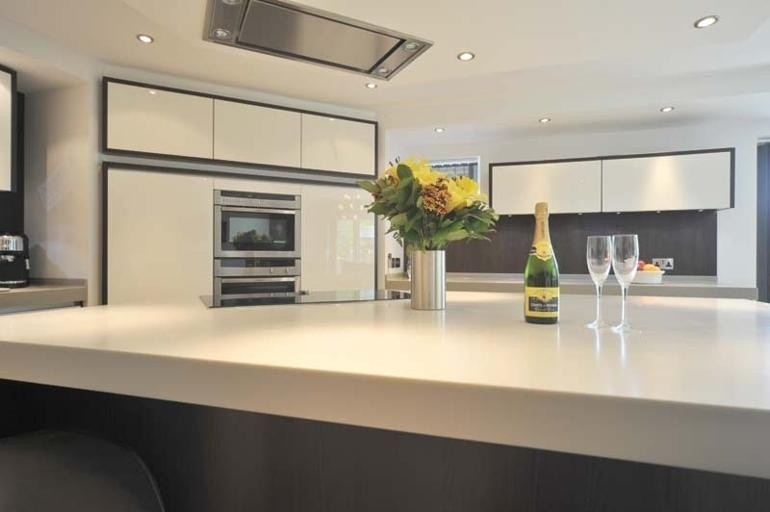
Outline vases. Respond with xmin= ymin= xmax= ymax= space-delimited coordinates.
xmin=412 ymin=250 xmax=446 ymax=310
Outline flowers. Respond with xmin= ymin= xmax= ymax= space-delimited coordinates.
xmin=360 ymin=156 xmax=499 ymax=248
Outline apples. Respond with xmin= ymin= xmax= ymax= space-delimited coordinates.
xmin=636 ymin=260 xmax=644 ymax=270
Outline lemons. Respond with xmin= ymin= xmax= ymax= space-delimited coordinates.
xmin=642 ymin=263 xmax=660 ymax=272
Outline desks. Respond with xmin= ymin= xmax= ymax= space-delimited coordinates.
xmin=0 ymin=280 xmax=88 ymax=311
xmin=386 ymin=269 xmax=716 ymax=296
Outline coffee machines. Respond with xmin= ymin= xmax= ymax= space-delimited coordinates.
xmin=0 ymin=232 xmax=31 ymax=288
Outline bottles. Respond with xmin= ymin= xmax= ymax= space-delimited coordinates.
xmin=525 ymin=202 xmax=561 ymax=322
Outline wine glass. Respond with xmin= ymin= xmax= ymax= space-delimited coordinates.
xmin=584 ymin=235 xmax=611 ymax=328
xmin=611 ymin=234 xmax=639 ymax=331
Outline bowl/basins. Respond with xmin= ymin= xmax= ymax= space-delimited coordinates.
xmin=633 ymin=270 xmax=665 ymax=285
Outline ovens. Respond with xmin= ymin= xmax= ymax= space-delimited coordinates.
xmin=213 ymin=189 xmax=309 ymax=305
